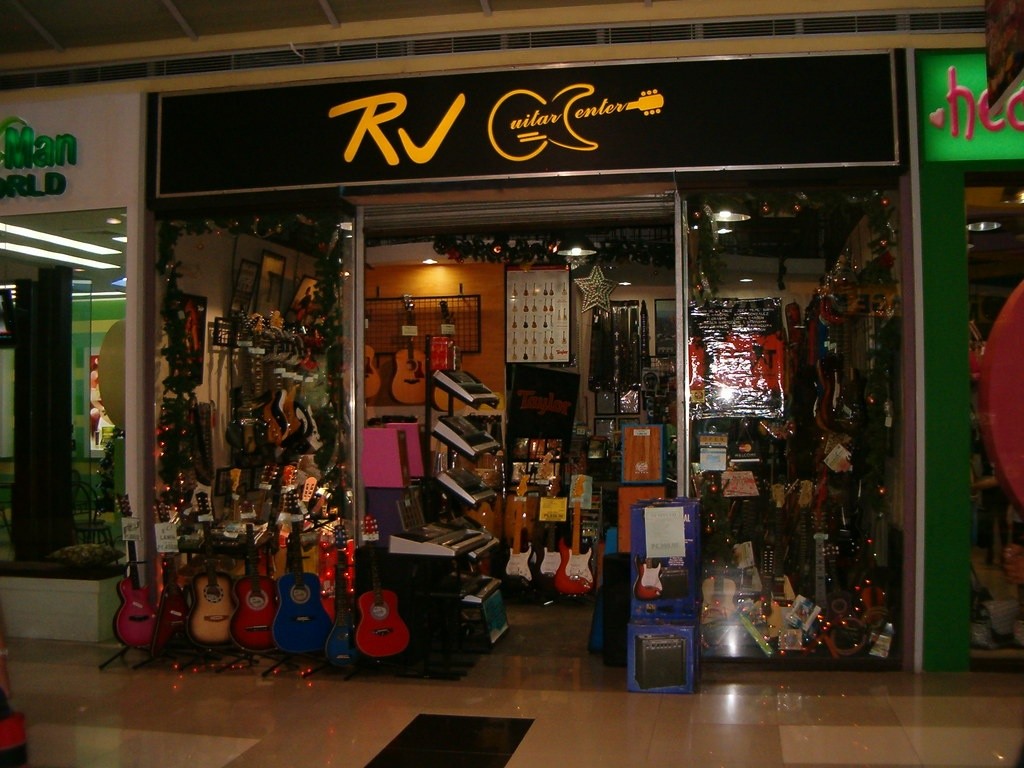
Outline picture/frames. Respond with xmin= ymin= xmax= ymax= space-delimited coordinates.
xmin=228 ymin=250 xmax=319 ymax=315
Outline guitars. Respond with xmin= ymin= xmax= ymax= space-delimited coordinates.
xmin=110 ymin=488 xmax=412 ymax=674
xmin=224 ymin=308 xmax=331 ymax=471
xmin=500 ymin=445 xmax=597 ymax=598
xmin=770 ymin=214 xmax=900 ymax=661
xmin=632 ymin=551 xmax=668 ymax=602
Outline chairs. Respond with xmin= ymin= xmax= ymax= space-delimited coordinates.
xmin=1 ymin=468 xmax=122 ymax=565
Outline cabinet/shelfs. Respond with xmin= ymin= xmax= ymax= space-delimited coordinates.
xmin=387 ymin=338 xmax=504 ymax=682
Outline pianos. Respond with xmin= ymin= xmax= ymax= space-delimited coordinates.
xmin=383 ymin=366 xmax=504 ymax=566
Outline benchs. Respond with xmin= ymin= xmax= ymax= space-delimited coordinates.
xmin=0 ymin=560 xmax=125 ymax=643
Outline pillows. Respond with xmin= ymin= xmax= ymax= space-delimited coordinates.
xmin=46 ymin=544 xmax=125 ymax=571
